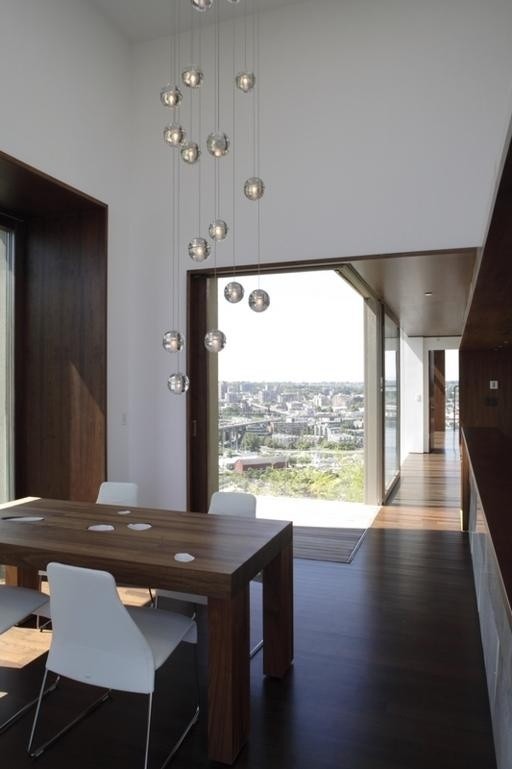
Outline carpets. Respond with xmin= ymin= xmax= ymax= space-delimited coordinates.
xmin=256 ymin=497 xmax=382 ymax=564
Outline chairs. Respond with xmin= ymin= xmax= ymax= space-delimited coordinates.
xmin=27 ymin=482 xmax=155 ymax=631
xmin=1 ymin=582 xmax=61 ymax=733
xmin=153 ymin=492 xmax=263 ymax=660
xmin=26 ymin=559 xmax=198 ymax=769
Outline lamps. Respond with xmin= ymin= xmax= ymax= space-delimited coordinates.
xmin=159 ymin=1 xmax=269 ymax=393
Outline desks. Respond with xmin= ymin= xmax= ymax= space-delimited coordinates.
xmin=0 ymin=496 xmax=292 ymax=765
